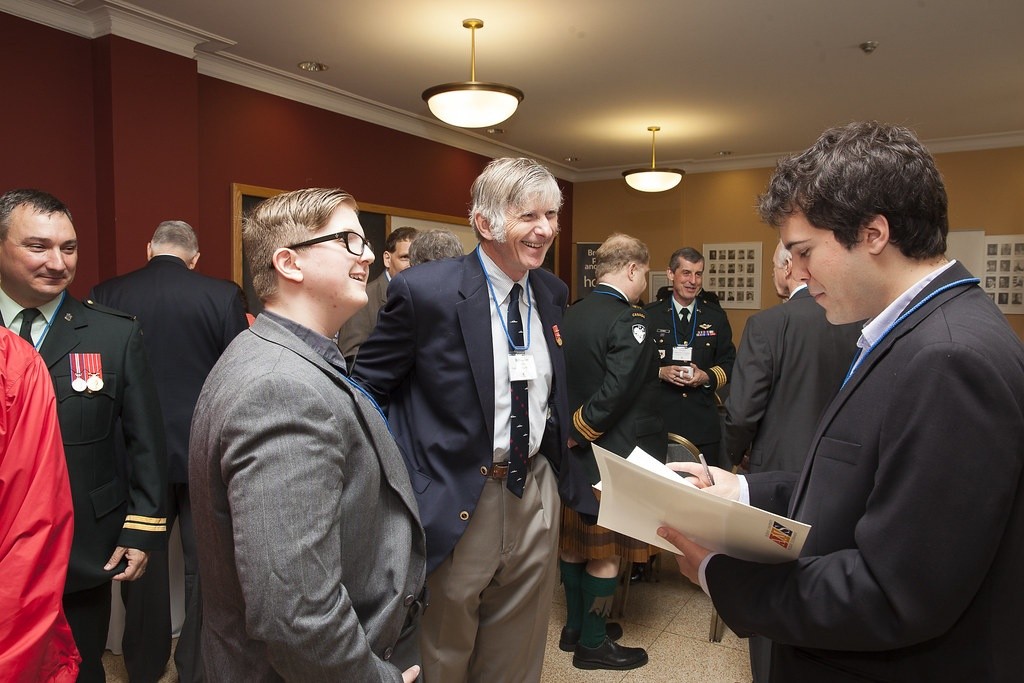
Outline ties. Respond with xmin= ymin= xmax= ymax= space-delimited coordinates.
xmin=680 ymin=307 xmax=691 ymax=342
xmin=507 ymin=284 xmax=529 ymax=500
xmin=19 ymin=308 xmax=41 ymax=347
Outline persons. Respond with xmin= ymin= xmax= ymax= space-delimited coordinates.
xmin=0 ymin=328 xmax=86 ymax=683
xmin=655 ymin=120 xmax=1024 ymax=683
xmin=556 ymin=232 xmax=661 ymax=671
xmin=630 ymin=247 xmax=735 ymax=583
xmin=709 ymin=250 xmax=755 ymax=301
xmin=337 ymin=226 xmax=465 ymax=356
xmin=349 ymin=157 xmax=569 ymax=683
xmin=174 ymin=186 xmax=428 ymax=683
xmin=985 ymin=243 xmax=1024 ymax=304
xmin=88 ymin=219 xmax=250 ymax=683
xmin=0 ymin=189 xmax=179 ymax=683
xmin=723 ymin=237 xmax=871 ymax=473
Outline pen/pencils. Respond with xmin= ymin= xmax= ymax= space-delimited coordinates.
xmin=698 ymin=454 xmax=716 ymax=486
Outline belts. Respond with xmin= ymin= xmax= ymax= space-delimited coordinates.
xmin=489 ymin=452 xmax=541 ymax=480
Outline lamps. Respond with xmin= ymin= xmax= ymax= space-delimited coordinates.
xmin=622 ymin=126 xmax=686 ymax=193
xmin=422 ymin=17 xmax=525 ymax=129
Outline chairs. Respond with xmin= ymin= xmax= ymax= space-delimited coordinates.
xmin=620 ymin=428 xmax=725 ymax=644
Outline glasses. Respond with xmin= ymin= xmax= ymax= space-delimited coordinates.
xmin=269 ymin=230 xmax=371 ymax=269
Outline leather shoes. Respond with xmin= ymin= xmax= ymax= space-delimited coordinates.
xmin=572 ymin=636 xmax=648 ymax=670
xmin=559 ymin=622 xmax=623 ymax=652
xmin=623 ymin=553 xmax=656 ymax=582
